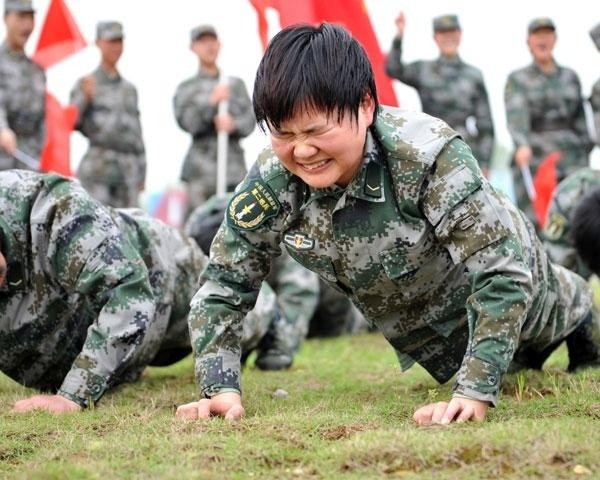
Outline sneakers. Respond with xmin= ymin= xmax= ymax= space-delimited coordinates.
xmin=256 ymin=308 xmax=297 ymax=370
xmin=568 ymin=310 xmax=600 ymax=376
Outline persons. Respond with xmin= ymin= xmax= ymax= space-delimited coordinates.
xmin=505 ymin=17 xmax=593 ymax=239
xmin=68 ymin=21 xmax=146 ymax=208
xmin=175 ymin=23 xmax=600 ymax=425
xmin=385 ymin=11 xmax=493 ymax=182
xmin=175 ymin=26 xmax=256 ymax=215
xmin=540 ymin=169 xmax=600 ymax=283
xmin=0 ymin=169 xmax=293 ymax=415
xmin=187 ymin=194 xmax=373 ymax=343
xmin=0 ymin=0 xmax=48 ymax=169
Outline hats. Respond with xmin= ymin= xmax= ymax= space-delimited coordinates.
xmin=190 ymin=25 xmax=217 ymax=42
xmin=2 ymin=0 xmax=35 ymax=15
xmin=96 ymin=22 xmax=124 ymax=42
xmin=433 ymin=15 xmax=460 ymax=33
xmin=529 ymin=18 xmax=554 ymax=33
